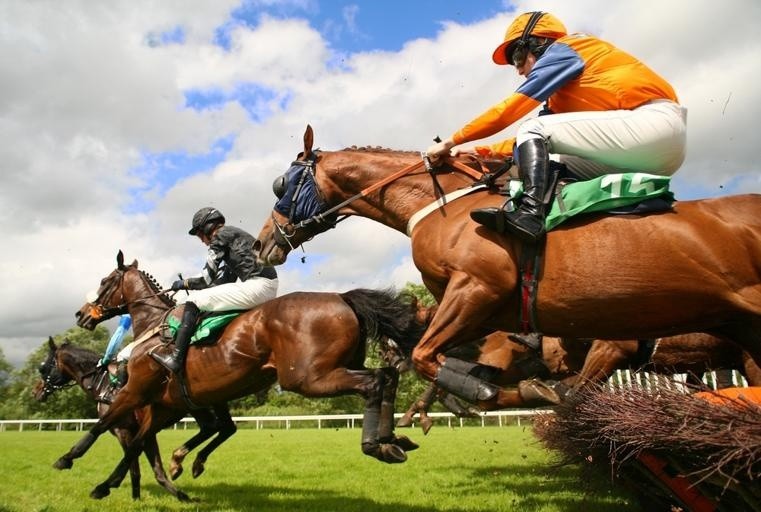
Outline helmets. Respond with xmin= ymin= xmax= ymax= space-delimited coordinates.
xmin=189 ymin=208 xmax=225 ymax=235
xmin=493 ymin=12 xmax=565 ymax=65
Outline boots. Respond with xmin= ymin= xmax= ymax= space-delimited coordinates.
xmin=96 ymin=362 xmax=127 ymax=401
xmin=471 ymin=139 xmax=549 ymax=242
xmin=148 ymin=311 xmax=197 ymax=375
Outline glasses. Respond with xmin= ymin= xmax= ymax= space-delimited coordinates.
xmin=513 ymin=12 xmax=542 ymax=67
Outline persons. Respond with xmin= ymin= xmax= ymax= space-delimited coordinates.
xmin=422 ymin=12 xmax=691 ymax=242
xmin=95 ymin=314 xmax=133 ymax=387
xmin=506 ymin=332 xmax=542 ymax=353
xmin=147 ymin=205 xmax=279 ymax=376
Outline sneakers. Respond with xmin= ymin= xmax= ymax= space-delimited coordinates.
xmin=507 ymin=333 xmax=542 ymax=351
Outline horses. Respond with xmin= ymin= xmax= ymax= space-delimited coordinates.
xmin=31 ymin=335 xmax=270 ymax=480
xmin=50 ymin=248 xmax=433 ymax=499
xmin=250 ymin=122 xmax=760 ymax=435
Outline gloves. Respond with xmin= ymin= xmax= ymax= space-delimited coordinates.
xmin=172 ymin=278 xmax=190 ymax=291
